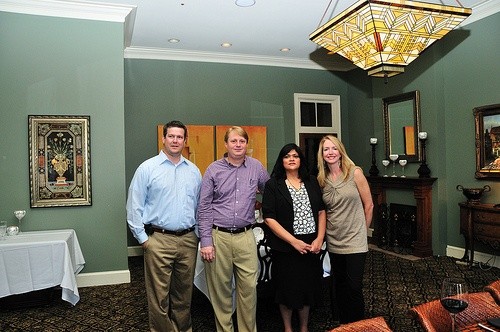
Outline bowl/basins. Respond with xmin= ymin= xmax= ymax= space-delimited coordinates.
xmin=6 ymin=226 xmax=19 ymax=236
xmin=457 ymin=184 xmax=490 ymax=204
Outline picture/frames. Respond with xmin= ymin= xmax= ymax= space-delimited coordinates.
xmin=27 ymin=114 xmax=93 ymax=209
xmin=382 ymin=90 xmax=424 ymax=164
xmin=472 ymin=103 xmax=500 ymax=182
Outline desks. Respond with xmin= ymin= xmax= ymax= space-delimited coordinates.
xmin=366 ymin=175 xmax=437 ymax=258
xmin=0 ymin=228 xmax=86 ymax=306
xmin=458 ymin=202 xmax=500 ymax=270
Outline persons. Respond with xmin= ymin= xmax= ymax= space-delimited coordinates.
xmin=262 ymin=143 xmax=326 ymax=332
xmin=126 ymin=121 xmax=202 ymax=332
xmin=198 ymin=125 xmax=270 ymax=332
xmin=317 ymin=135 xmax=374 ymax=327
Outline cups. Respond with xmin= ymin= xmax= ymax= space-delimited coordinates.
xmin=0 ymin=221 xmax=7 ymax=240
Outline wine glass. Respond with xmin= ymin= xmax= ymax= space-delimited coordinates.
xmin=14 ymin=210 xmax=26 ymax=236
xmin=382 ymin=155 xmax=406 ymax=178
xmin=441 ymin=277 xmax=469 ymax=332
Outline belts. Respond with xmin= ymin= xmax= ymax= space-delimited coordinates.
xmin=212 ymin=224 xmax=252 ymax=234
xmin=151 ymin=227 xmax=195 ymax=236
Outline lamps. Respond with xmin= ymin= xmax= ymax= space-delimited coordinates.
xmin=308 ymin=0 xmax=473 ymax=85
